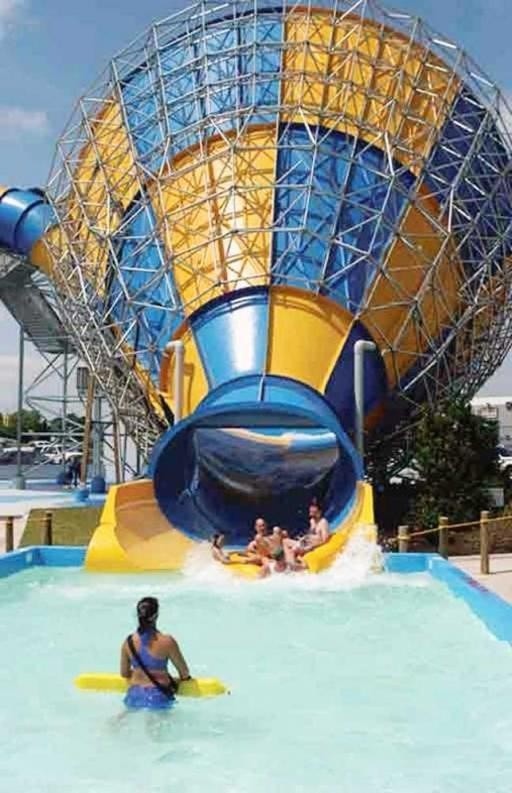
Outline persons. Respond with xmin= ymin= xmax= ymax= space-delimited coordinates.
xmin=208 ymin=531 xmax=262 ymax=566
xmin=247 ymin=496 xmax=331 ymax=575
xmin=119 ymin=595 xmax=191 ymax=711
